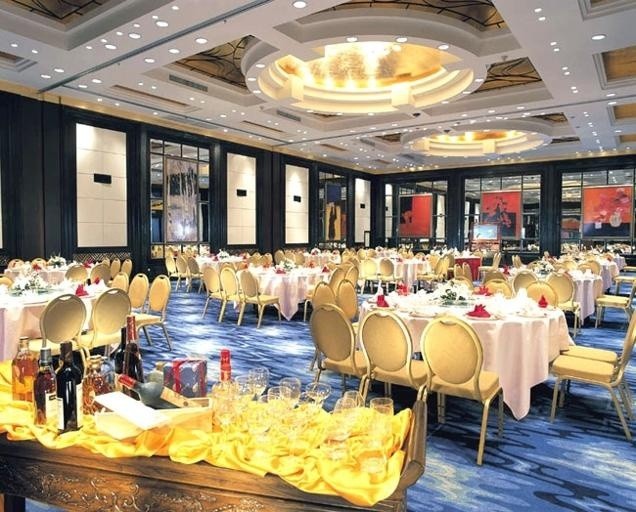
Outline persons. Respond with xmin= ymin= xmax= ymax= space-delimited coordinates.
xmin=325 ymin=205 xmax=338 ymax=241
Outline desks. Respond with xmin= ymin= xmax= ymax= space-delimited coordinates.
xmin=0 ymin=358 xmax=429 ymax=511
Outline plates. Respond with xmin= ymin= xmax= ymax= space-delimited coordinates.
xmin=368 ymin=292 xmax=556 ymax=321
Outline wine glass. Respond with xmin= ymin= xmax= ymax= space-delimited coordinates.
xmin=210 ymin=366 xmax=397 ymax=459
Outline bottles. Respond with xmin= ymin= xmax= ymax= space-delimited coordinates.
xmin=219 ymin=348 xmax=232 ymax=383
xmin=114 ymin=316 xmax=143 ymax=400
xmin=13 ymin=335 xmax=111 ymax=434
xmin=117 ymin=372 xmax=204 ymax=411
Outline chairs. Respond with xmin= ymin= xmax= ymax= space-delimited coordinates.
xmin=0 ymin=256 xmax=173 ymax=369
